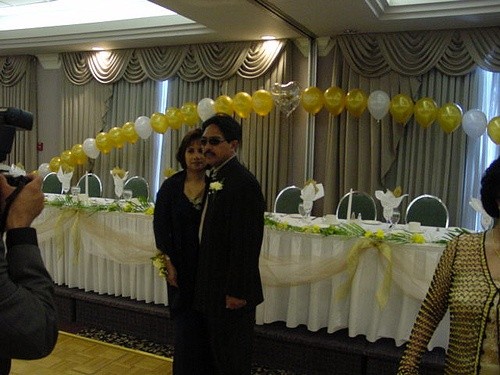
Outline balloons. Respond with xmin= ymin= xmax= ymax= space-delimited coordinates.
xmin=29 ymin=80 xmax=500 ymax=177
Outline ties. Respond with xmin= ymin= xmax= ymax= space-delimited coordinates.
xmin=199 ymin=170 xmax=214 ymax=246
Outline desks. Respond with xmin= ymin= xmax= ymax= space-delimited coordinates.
xmin=31 ymin=193 xmax=476 ymax=354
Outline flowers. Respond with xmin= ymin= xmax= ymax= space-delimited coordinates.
xmin=150 ymin=253 xmax=168 ymax=277
xmin=210 ymin=181 xmax=223 ymax=195
xmin=44 ymin=196 xmax=472 ymax=245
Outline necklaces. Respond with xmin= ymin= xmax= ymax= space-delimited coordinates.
xmin=492 ymin=229 xmax=500 ymax=257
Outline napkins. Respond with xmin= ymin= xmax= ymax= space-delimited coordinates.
xmin=110 ymin=170 xmax=129 ymax=197
xmin=300 ymin=183 xmax=324 ymax=209
xmin=375 ymin=189 xmax=409 ymax=218
xmin=57 ymin=165 xmax=73 ymax=189
xmin=469 ymin=195 xmax=491 ymax=226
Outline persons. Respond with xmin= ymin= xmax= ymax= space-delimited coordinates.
xmin=397 ymin=157 xmax=500 ymax=375
xmin=151 ymin=111 xmax=266 ymax=375
xmin=0 ymin=171 xmax=59 ymax=375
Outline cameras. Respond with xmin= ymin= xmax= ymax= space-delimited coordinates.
xmin=0 ymin=106 xmax=33 ymax=187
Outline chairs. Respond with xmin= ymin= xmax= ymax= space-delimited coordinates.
xmin=123 ymin=176 xmax=149 ymax=201
xmin=274 ymin=185 xmax=303 ymax=215
xmin=77 ymin=173 xmax=102 ymax=198
xmin=41 ymin=172 xmax=63 ymax=194
xmin=405 ymin=194 xmax=450 ymax=228
xmin=336 ymin=191 xmax=377 ymax=221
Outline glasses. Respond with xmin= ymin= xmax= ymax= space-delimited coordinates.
xmin=200 ymin=137 xmax=226 ymax=146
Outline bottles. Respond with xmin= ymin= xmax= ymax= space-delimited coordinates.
xmin=352 ymin=211 xmax=361 ymax=221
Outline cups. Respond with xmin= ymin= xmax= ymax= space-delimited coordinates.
xmin=408 ymin=222 xmax=420 ymax=231
xmin=48 ymin=194 xmax=57 ymax=202
xmin=481 ymin=214 xmax=494 ymax=231
xmin=326 ymin=215 xmax=337 ymax=223
xmin=71 ymin=188 xmax=80 ymax=197
xmin=78 ymin=194 xmax=88 ymax=201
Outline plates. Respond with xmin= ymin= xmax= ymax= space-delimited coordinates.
xmin=404 ymin=226 xmax=425 ymax=233
xmin=362 ymin=221 xmax=382 ymax=224
xmin=322 ymin=220 xmax=343 ymax=225
xmin=271 ymin=213 xmax=303 ymax=218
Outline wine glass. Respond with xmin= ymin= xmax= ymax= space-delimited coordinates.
xmin=384 ymin=208 xmax=400 ymax=230
xmin=114 ymin=186 xmax=132 ymax=205
xmin=63 ymin=182 xmax=70 ymax=194
xmin=298 ymin=201 xmax=313 ymax=225
